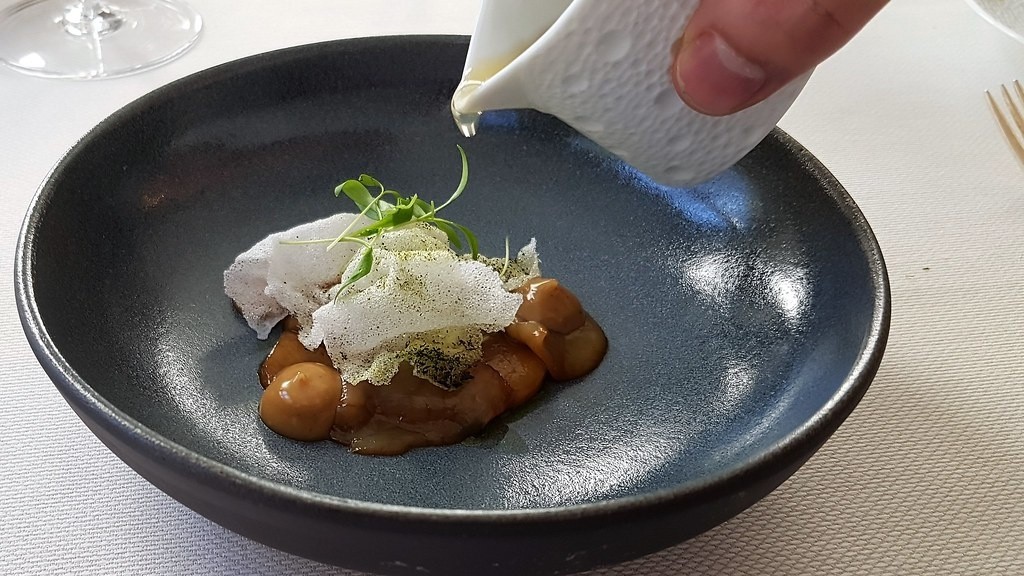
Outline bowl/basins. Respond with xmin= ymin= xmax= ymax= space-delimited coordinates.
xmin=14 ymin=33 xmax=892 ymax=576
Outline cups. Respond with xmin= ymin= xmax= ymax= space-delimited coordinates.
xmin=450 ymin=0 xmax=814 ymax=194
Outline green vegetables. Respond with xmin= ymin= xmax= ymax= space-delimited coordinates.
xmin=275 ymin=144 xmax=478 ymax=306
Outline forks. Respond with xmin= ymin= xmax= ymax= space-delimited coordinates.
xmin=982 ymin=77 xmax=1024 ymax=164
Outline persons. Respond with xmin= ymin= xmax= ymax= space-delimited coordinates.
xmin=672 ymin=0 xmax=886 ymax=119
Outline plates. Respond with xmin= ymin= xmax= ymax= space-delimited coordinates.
xmin=964 ymin=0 xmax=1024 ymax=46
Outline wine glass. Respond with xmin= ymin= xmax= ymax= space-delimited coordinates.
xmin=0 ymin=0 xmax=204 ymax=82
